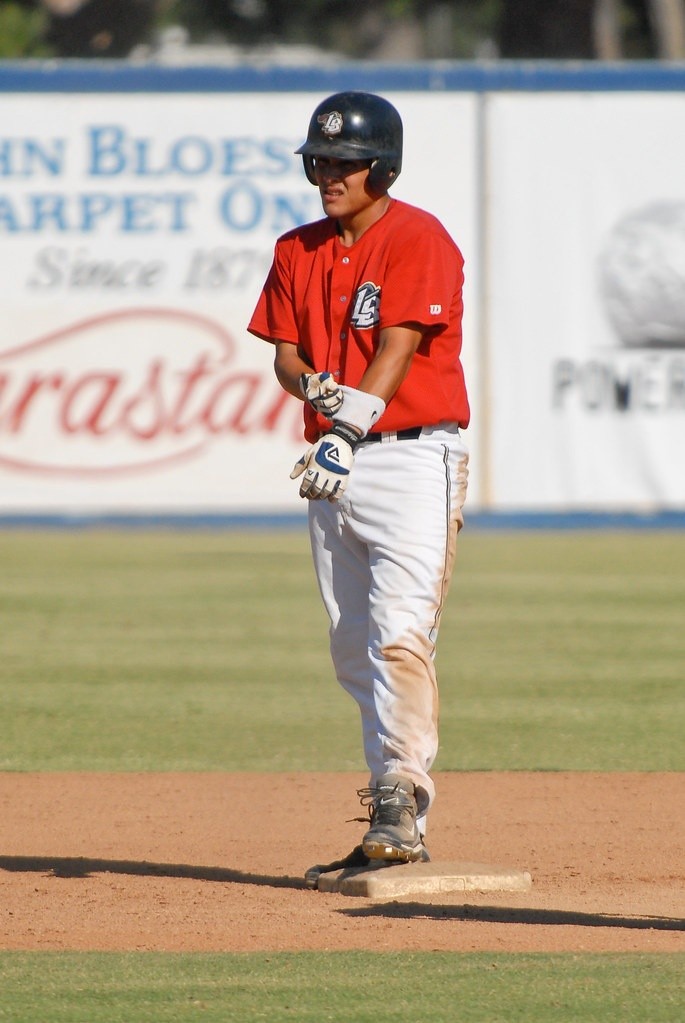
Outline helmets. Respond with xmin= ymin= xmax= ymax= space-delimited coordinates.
xmin=294 ymin=92 xmax=404 ymax=195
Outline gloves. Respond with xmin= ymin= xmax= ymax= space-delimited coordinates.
xmin=299 ymin=371 xmax=343 ymax=417
xmin=290 ymin=422 xmax=361 ymax=502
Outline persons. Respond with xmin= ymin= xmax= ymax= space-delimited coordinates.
xmin=245 ymin=90 xmax=471 ymax=886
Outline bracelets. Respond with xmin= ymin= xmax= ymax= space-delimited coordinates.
xmin=326 ymin=384 xmax=387 ymax=437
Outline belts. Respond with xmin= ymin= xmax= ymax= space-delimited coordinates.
xmin=361 ymin=428 xmax=422 ymax=442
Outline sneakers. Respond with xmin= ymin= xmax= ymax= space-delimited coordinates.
xmin=305 ymin=804 xmax=430 ymax=889
xmin=357 ymin=774 xmax=423 ymax=863
